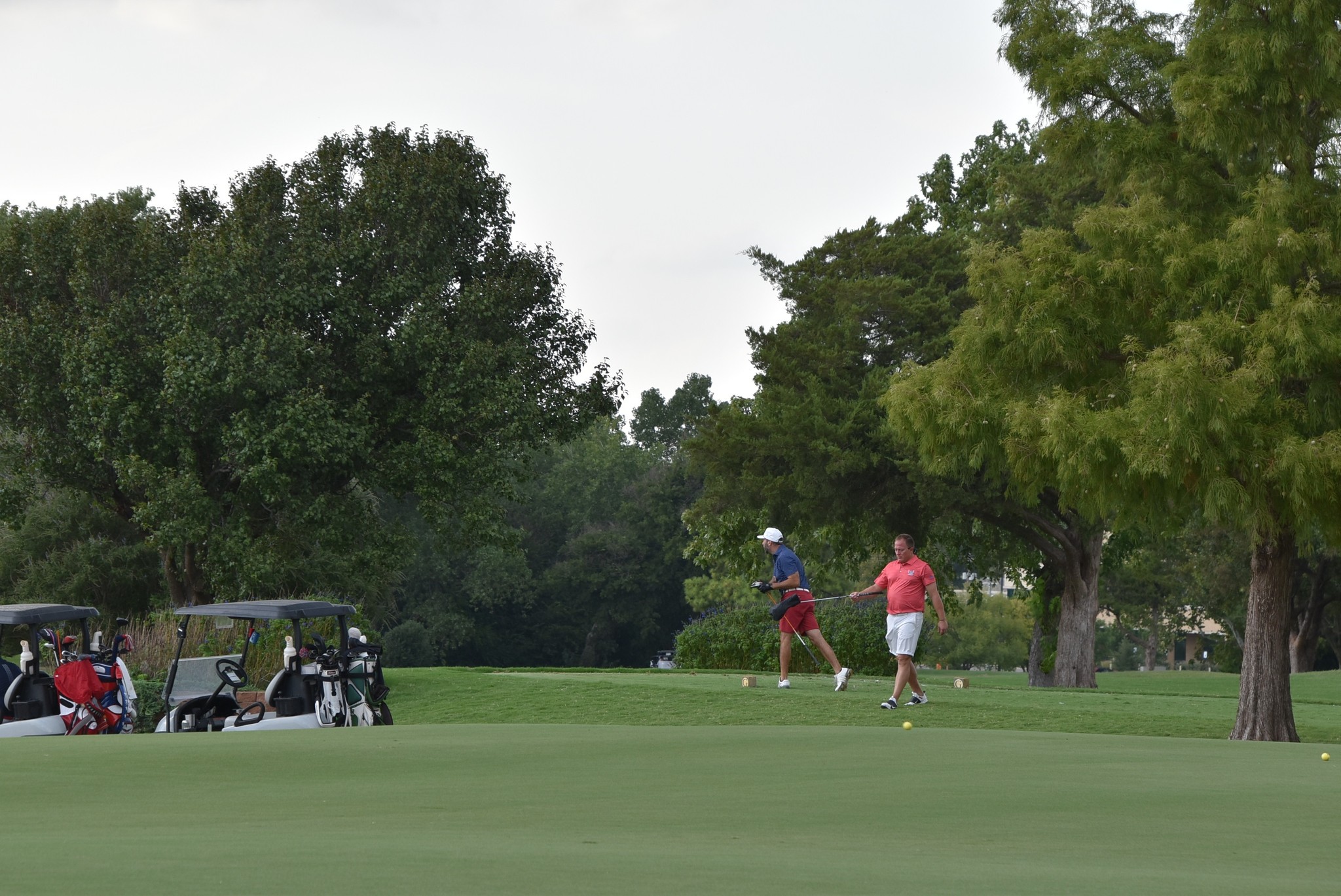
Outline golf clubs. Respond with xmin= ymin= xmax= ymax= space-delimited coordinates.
xmin=39 ymin=616 xmax=130 ymax=667
xmin=801 ymin=591 xmax=884 ymax=603
xmin=750 ymin=580 xmax=819 ymax=665
xmin=305 ymin=642 xmax=348 ymax=665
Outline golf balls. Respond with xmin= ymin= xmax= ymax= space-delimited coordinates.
xmin=1321 ymin=753 xmax=1330 ymax=760
xmin=903 ymin=722 xmax=912 ymax=730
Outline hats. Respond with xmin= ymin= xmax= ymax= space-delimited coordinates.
xmin=757 ymin=528 xmax=784 ymax=543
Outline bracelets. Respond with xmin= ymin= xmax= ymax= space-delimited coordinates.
xmin=940 ymin=618 xmax=946 ymax=622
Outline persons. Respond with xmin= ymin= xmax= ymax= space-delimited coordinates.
xmin=849 ymin=534 xmax=949 ymax=710
xmin=752 ymin=528 xmax=853 ymax=692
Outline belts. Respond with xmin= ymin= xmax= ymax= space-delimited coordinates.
xmin=893 ymin=611 xmax=922 ymax=616
xmin=783 ymin=587 xmax=810 ymax=595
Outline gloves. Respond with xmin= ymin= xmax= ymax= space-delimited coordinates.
xmin=757 ymin=583 xmax=773 ymax=594
xmin=750 ymin=580 xmax=767 ymax=588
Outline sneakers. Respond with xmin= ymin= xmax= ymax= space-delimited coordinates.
xmin=834 ymin=667 xmax=853 ymax=691
xmin=881 ymin=695 xmax=898 ymax=710
xmin=778 ymin=676 xmax=790 ymax=688
xmin=904 ymin=691 xmax=928 ymax=706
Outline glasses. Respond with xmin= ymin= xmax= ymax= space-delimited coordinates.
xmin=908 ymin=547 xmax=917 ymax=554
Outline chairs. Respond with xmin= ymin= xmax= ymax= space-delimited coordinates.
xmin=224 ymin=668 xmax=287 ymax=728
xmin=2 ymin=670 xmax=50 ymax=723
